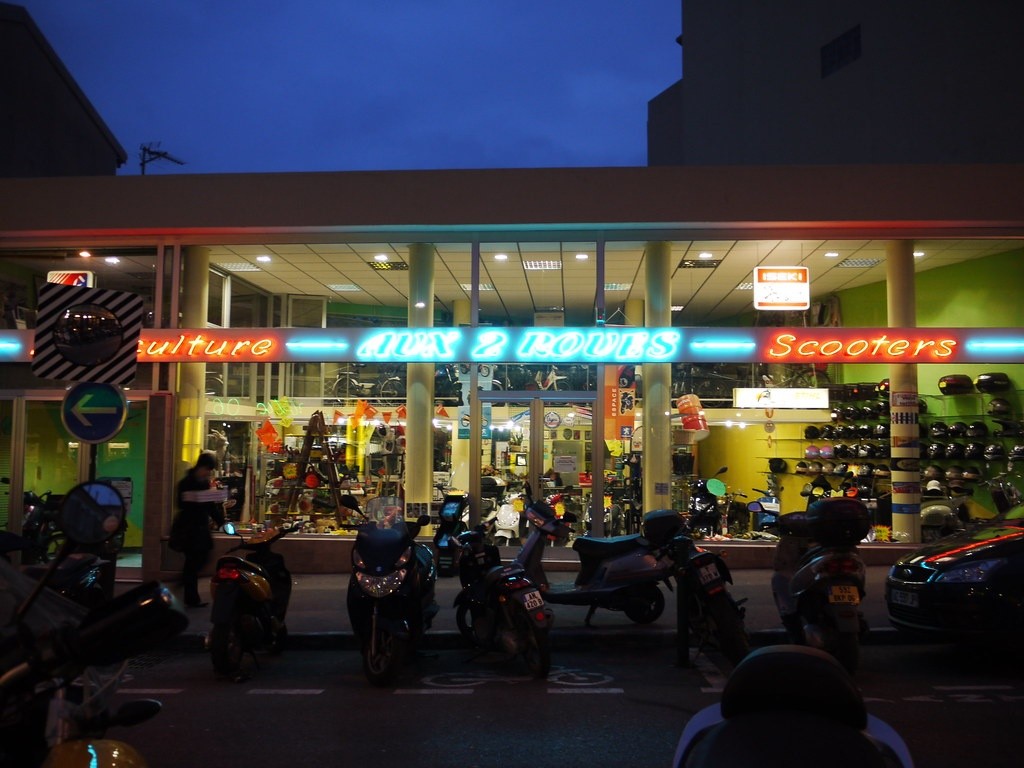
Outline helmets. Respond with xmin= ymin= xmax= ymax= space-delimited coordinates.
xmin=988 ymin=399 xmax=1011 ymax=416
xmin=797 ymin=399 xmax=1006 ymax=484
xmin=769 ymin=459 xmax=787 ymax=473
xmin=1008 ymin=444 xmax=1024 ymax=460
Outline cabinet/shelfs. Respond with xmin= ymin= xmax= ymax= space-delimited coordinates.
xmin=265 ymin=408 xmax=360 ymax=527
xmin=581 ymin=440 xmax=625 ymax=489
xmin=755 ymin=388 xmax=1024 ymax=483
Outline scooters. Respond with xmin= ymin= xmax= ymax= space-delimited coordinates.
xmin=367 ymin=320 xmax=766 ymax=548
xmin=752 ymin=488 xmax=782 ymax=538
xmin=338 ymin=495 xmax=439 ymax=681
xmin=0 ymin=480 xmax=191 ymax=768
xmin=510 ymin=482 xmax=686 ymax=623
xmin=679 ymin=464 xmax=728 ymax=536
xmin=652 ymin=486 xmax=752 ymax=670
xmin=919 ymin=465 xmax=1022 ymax=540
xmin=447 ymin=519 xmax=551 ymax=674
xmin=209 ymin=520 xmax=316 ymax=677
xmin=746 ymin=498 xmax=873 ymax=672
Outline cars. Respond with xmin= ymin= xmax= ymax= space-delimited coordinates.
xmin=885 ymin=496 xmax=1024 ymax=651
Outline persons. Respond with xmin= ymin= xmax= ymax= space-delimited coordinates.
xmin=550 ymin=472 xmax=563 ymax=487
xmin=625 ymin=455 xmax=641 ymax=499
xmin=165 ymin=450 xmax=226 ymax=609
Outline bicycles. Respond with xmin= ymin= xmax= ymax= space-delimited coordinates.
xmin=333 ymin=364 xmax=406 ymax=407
xmin=753 ymin=363 xmax=831 ymax=395
xmin=206 ymin=370 xmax=225 ymax=399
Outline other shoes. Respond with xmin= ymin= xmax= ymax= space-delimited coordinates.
xmin=186 ymin=600 xmax=210 ymax=607
xmin=86 ymin=699 xmax=162 ymax=732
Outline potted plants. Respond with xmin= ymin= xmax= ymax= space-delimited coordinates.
xmin=508 ymin=434 xmax=524 ymax=452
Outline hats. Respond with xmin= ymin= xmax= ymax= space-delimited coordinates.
xmin=927 ymin=480 xmax=942 ymax=492
xmin=945 ymin=480 xmax=968 ymax=491
